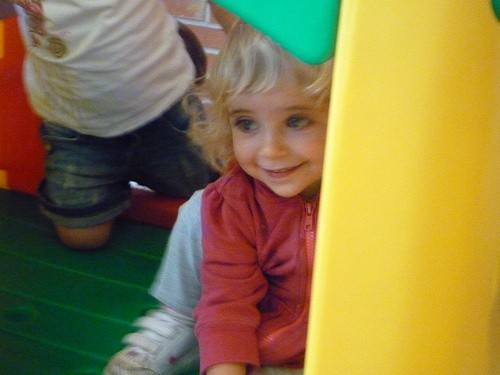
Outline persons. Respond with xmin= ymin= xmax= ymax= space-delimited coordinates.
xmin=193 ymin=15 xmax=335 ymax=375
xmin=12 ymin=0 xmax=235 ymax=250
xmin=104 ymin=188 xmax=204 ymax=375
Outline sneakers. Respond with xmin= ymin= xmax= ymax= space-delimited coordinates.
xmin=104 ymin=306 xmax=202 ymax=375
